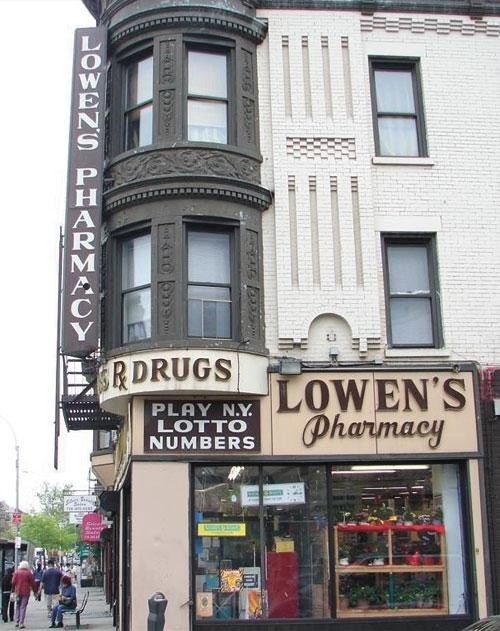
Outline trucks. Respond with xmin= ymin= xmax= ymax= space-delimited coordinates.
xmin=35 ymin=547 xmax=48 ymax=564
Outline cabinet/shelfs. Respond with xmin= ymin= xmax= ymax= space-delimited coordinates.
xmin=334 ymin=525 xmax=449 ymax=615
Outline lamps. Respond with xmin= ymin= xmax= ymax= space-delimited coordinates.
xmin=279 ymin=359 xmax=302 ymax=375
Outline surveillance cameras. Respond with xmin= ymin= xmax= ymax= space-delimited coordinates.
xmin=330 ymin=347 xmax=339 ymax=365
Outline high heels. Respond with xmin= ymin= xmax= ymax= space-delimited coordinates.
xmin=49 ymin=623 xmax=56 ymax=627
xmin=56 ymin=622 xmax=63 ymax=627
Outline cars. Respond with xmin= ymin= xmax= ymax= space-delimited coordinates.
xmin=340 ymin=555 xmax=443 ymax=581
xmin=458 ymin=614 xmax=500 ymax=631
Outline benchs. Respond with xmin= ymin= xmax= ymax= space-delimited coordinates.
xmin=63 ymin=591 xmax=89 ymax=630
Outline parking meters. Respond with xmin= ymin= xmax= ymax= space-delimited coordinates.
xmin=146 ymin=592 xmax=168 ymax=631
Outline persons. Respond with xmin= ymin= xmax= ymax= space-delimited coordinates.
xmin=32 ymin=561 xmax=73 ymax=602
xmin=34 ymin=559 xmax=65 ymax=616
xmin=1 ymin=567 xmax=15 ymax=622
xmin=48 ymin=575 xmax=77 ymax=629
xmin=10 ymin=560 xmax=38 ymax=629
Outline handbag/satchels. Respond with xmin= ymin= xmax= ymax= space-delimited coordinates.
xmin=60 ymin=598 xmax=74 ymax=607
xmin=10 ymin=585 xmax=20 ymax=602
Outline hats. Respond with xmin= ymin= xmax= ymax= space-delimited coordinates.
xmin=20 ymin=560 xmax=28 ymax=569
